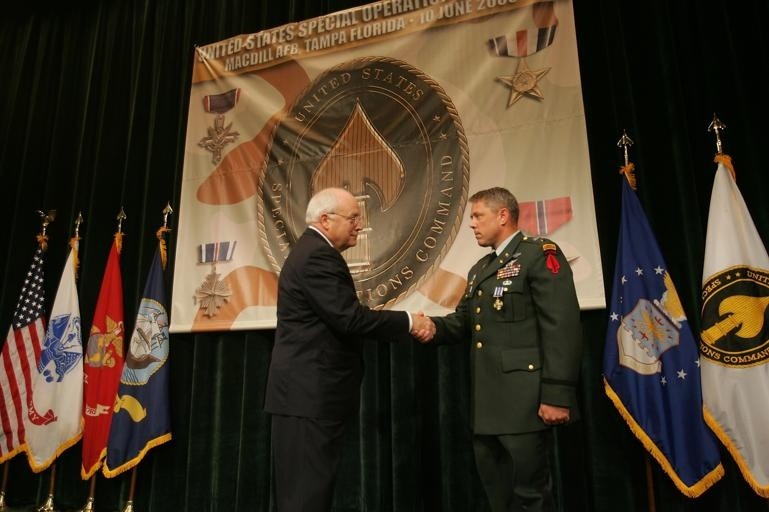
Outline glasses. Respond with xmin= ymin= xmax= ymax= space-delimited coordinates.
xmin=327 ymin=211 xmax=360 ymax=225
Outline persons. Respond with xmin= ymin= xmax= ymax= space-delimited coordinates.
xmin=411 ymin=187 xmax=584 ymax=511
xmin=263 ymin=186 xmax=437 ymax=511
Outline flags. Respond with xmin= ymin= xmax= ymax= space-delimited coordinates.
xmin=24 ymin=235 xmax=85 ymax=476
xmin=0 ymin=236 xmax=48 ymax=466
xmin=79 ymin=232 xmax=126 ymax=482
xmin=600 ymin=165 xmax=727 ymax=499
xmin=102 ymin=227 xmax=174 ymax=480
xmin=168 ymin=0 xmax=607 ymax=334
xmin=698 ymin=153 xmax=769 ymax=499
